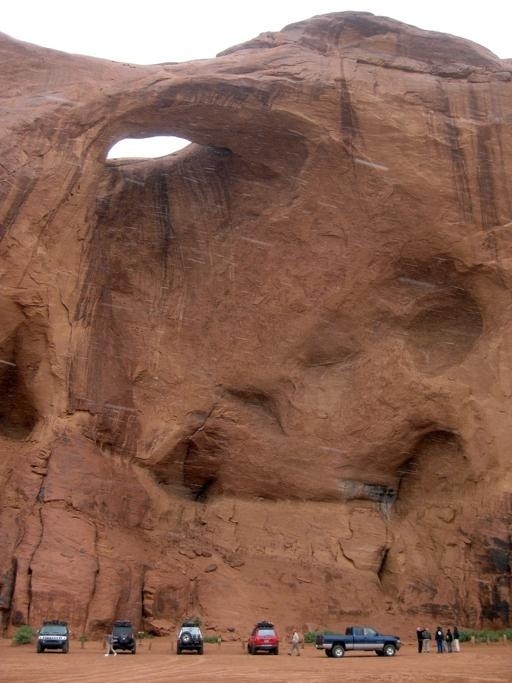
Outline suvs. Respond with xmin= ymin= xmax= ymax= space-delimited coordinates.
xmin=247 ymin=626 xmax=279 ymax=655
xmin=108 ymin=627 xmax=136 ymax=654
xmin=177 ymin=627 xmax=204 ymax=655
xmin=36 ymin=624 xmax=69 ymax=653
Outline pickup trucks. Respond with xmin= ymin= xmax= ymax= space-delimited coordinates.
xmin=315 ymin=625 xmax=401 ymax=657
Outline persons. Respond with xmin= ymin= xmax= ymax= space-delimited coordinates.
xmin=453 ymin=625 xmax=461 ymax=652
xmin=434 ymin=625 xmax=444 ymax=653
xmin=440 ymin=626 xmax=446 ymax=651
xmin=422 ymin=626 xmax=433 ymax=653
xmin=446 ymin=628 xmax=454 ymax=652
xmin=416 ymin=626 xmax=427 ymax=653
xmin=102 ymin=630 xmax=117 ymax=656
xmin=287 ymin=627 xmax=301 ymax=656
